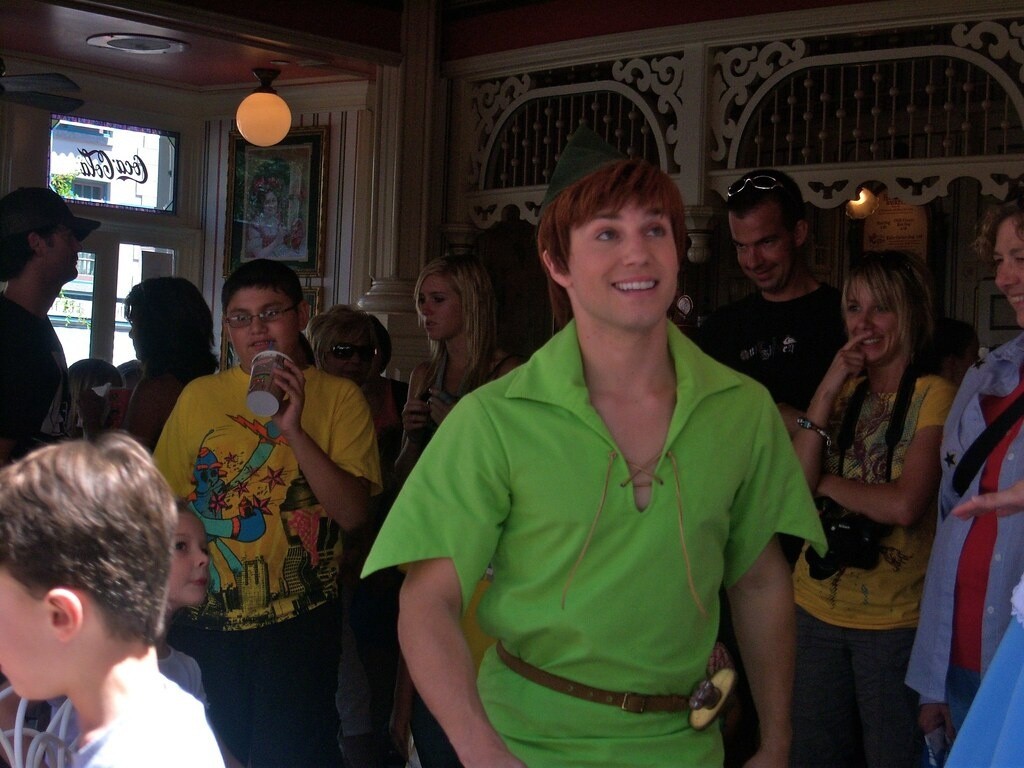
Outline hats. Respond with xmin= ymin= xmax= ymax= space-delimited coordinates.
xmin=0 ymin=187 xmax=101 ymax=241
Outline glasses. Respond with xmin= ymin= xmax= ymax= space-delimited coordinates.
xmin=222 ymin=304 xmax=294 ymax=328
xmin=331 ymin=341 xmax=374 ymax=361
xmin=728 ymin=175 xmax=784 ymax=197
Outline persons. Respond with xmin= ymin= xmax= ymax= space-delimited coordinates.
xmin=788 ymin=252 xmax=959 ymax=768
xmin=361 ymin=154 xmax=828 ymax=768
xmin=0 ymin=169 xmax=980 ymax=768
xmin=149 ymin=259 xmax=383 ymax=768
xmin=0 ymin=433 xmax=226 ymax=768
xmin=905 ymin=198 xmax=1024 ymax=768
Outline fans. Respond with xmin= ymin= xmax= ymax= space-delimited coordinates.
xmin=0 ymin=57 xmax=85 ymax=113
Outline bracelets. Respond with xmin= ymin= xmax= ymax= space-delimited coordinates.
xmin=794 ymin=416 xmax=831 ymax=450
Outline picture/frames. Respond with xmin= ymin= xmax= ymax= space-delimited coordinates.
xmin=974 ymin=277 xmax=1024 ymax=348
xmin=220 ymin=286 xmax=321 ymax=373
xmin=222 ymin=126 xmax=330 ymax=279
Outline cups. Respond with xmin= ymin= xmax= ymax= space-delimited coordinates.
xmin=246 ymin=351 xmax=293 ymax=417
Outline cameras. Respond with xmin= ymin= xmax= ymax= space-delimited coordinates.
xmin=805 ymin=509 xmax=879 ymax=580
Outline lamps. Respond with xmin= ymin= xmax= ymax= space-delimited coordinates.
xmin=844 ymin=187 xmax=881 ymax=222
xmin=235 ymin=68 xmax=292 ymax=147
